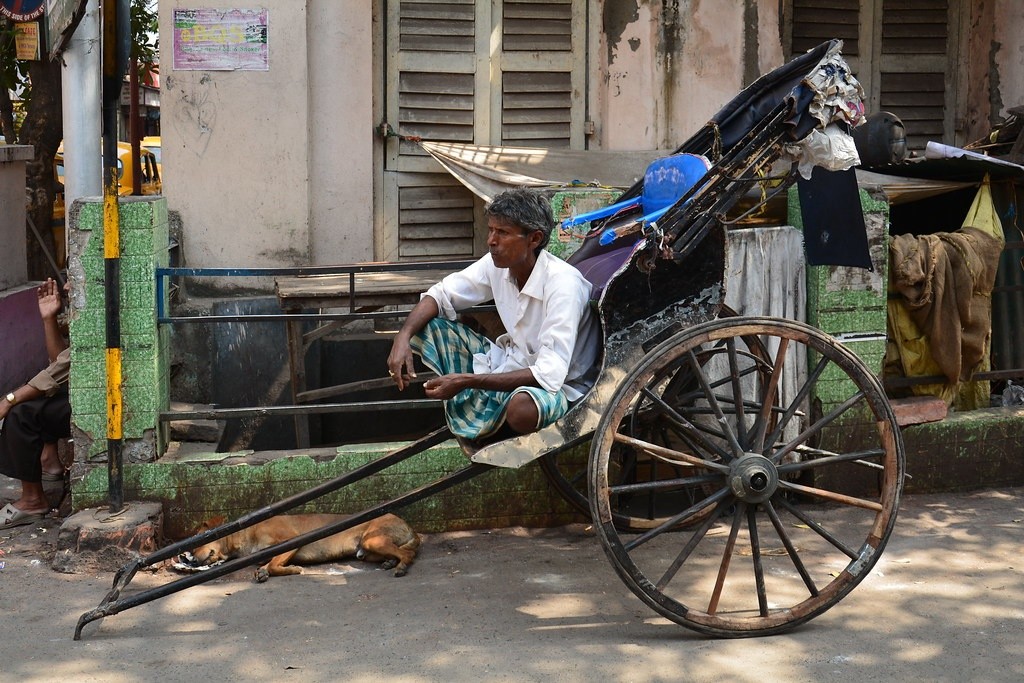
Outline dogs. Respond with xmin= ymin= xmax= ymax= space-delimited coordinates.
xmin=193 ymin=511 xmax=421 ymax=583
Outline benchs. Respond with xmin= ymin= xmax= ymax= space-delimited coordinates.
xmin=569 ymin=232 xmax=686 ymax=366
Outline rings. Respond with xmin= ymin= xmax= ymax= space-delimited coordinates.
xmin=389 ymin=370 xmax=395 ymax=377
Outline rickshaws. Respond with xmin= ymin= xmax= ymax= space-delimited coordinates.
xmin=73 ymin=38 xmax=905 ymax=639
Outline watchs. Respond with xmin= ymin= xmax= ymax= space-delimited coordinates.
xmin=6 ymin=392 xmax=17 ymax=405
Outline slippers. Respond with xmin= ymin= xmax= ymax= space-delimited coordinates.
xmin=0 ymin=502 xmax=48 ymax=529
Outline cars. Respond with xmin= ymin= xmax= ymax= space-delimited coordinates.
xmin=1 ymin=137 xmax=163 ymax=272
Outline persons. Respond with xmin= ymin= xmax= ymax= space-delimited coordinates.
xmin=387 ymin=188 xmax=602 ymax=447
xmin=0 ymin=256 xmax=70 ymax=530
